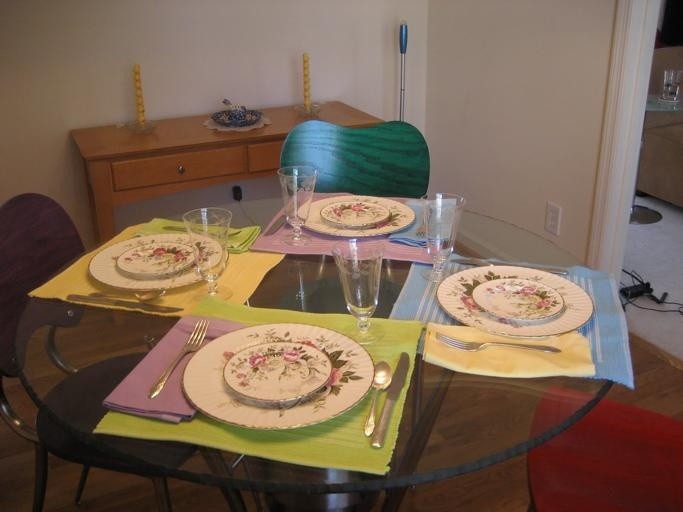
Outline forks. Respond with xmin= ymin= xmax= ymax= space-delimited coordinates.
xmin=432 ymin=330 xmax=562 ymax=356
xmin=160 ymin=224 xmax=241 ymax=238
xmin=144 ymin=318 xmax=211 ymax=399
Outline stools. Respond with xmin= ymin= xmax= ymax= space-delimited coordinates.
xmin=525 ymin=382 xmax=681 ymax=512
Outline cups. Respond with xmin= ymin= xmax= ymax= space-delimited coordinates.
xmin=661 ymin=69 xmax=682 ymax=102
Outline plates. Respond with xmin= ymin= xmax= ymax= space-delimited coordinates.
xmin=114 ymin=241 xmax=198 ymax=277
xmin=318 ymin=200 xmax=390 ymax=228
xmin=436 ymin=265 xmax=595 ymax=337
xmin=85 ymin=233 xmax=223 ymax=291
xmin=181 ymin=320 xmax=376 ymax=434
xmin=210 ymin=108 xmax=263 ymax=126
xmin=471 ymin=278 xmax=566 ymax=324
xmin=222 ymin=341 xmax=335 ymax=401
xmin=297 ymin=195 xmax=416 ymax=239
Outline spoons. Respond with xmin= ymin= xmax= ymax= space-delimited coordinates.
xmin=84 ymin=290 xmax=165 ymax=301
xmin=362 ymin=359 xmax=392 ymax=437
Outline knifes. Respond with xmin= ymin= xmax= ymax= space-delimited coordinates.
xmin=65 ymin=292 xmax=185 ymax=312
xmin=370 ymin=351 xmax=409 ymax=449
xmin=264 ymin=200 xmax=299 ymax=236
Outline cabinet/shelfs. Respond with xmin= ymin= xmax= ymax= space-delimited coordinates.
xmin=66 ymin=101 xmax=386 ymax=246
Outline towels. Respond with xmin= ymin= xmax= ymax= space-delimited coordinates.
xmin=416 ymin=317 xmax=597 ymax=383
xmin=389 ymin=198 xmax=457 ymax=246
xmin=133 ymin=214 xmax=261 ymax=256
xmin=100 ymin=313 xmax=240 ymax=425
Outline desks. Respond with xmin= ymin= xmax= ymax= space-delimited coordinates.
xmin=625 ymin=93 xmax=683 ymax=226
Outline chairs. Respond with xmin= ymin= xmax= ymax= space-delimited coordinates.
xmin=628 ymin=46 xmax=683 ymax=209
xmin=276 ymin=117 xmax=434 ymax=429
xmin=0 ymin=189 xmax=244 ymax=512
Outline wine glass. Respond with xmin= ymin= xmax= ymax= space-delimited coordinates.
xmin=418 ymin=192 xmax=466 ymax=283
xmin=181 ymin=206 xmax=234 ymax=302
xmin=275 ymin=163 xmax=319 ymax=248
xmin=329 ymin=237 xmax=386 ymax=350
